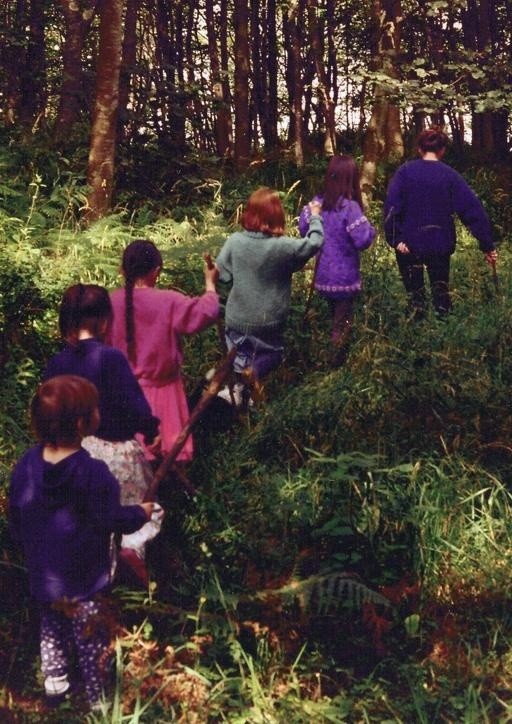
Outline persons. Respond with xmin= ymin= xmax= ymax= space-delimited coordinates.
xmin=42 ymin=283 xmax=162 ymax=591
xmin=102 ymin=241 xmax=221 ymax=463
xmin=215 ymin=187 xmax=325 ymax=376
xmin=8 ymin=374 xmax=154 ymax=708
xmin=384 ymin=128 xmax=498 ymax=319
xmin=299 ymin=157 xmax=376 ymax=366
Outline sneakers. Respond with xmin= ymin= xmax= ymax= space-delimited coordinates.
xmin=119 ymin=549 xmax=149 ymax=587
xmin=217 ymin=385 xmax=254 ymax=406
